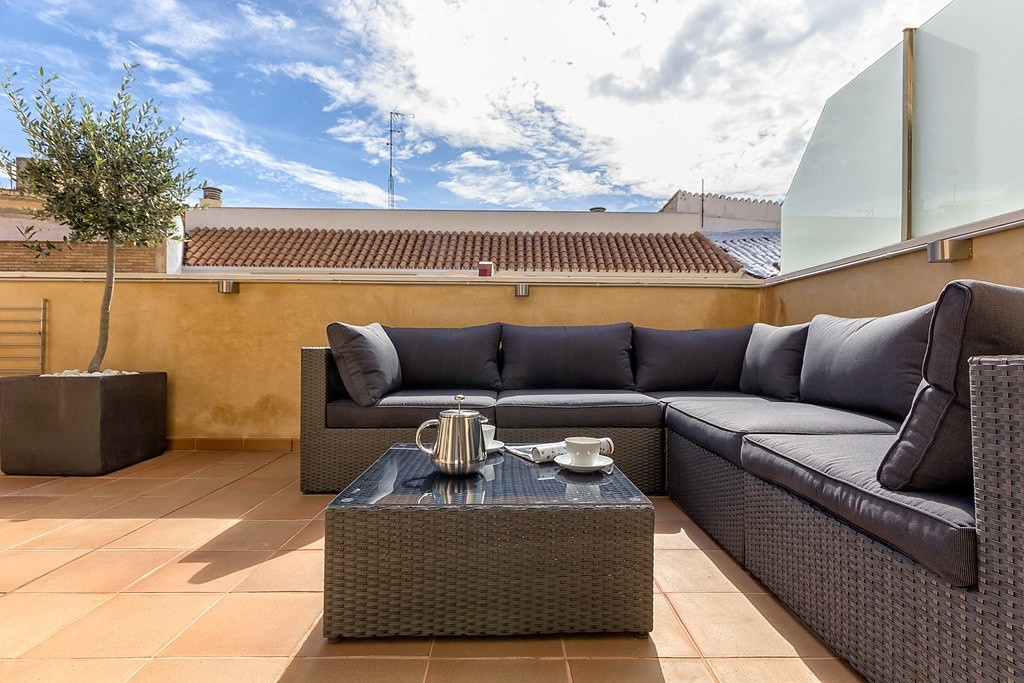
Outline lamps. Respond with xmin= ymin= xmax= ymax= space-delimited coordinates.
xmin=515 ymin=284 xmax=529 ymax=296
xmin=218 ymin=278 xmax=240 ymax=294
xmin=926 ymin=238 xmax=975 ymax=263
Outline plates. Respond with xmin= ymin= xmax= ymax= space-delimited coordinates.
xmin=486 ymin=440 xmax=505 ymax=454
xmin=554 ymin=454 xmax=613 ymax=473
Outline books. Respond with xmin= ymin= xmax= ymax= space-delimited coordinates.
xmin=504 ymin=437 xmax=614 ymax=464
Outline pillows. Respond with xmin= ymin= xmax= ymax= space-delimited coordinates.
xmin=503 ymin=321 xmax=635 ymax=393
xmin=382 ymin=321 xmax=502 ymax=391
xmin=797 ymin=300 xmax=937 ymax=418
xmin=876 ymin=279 xmax=1024 ymax=492
xmin=741 ymin=322 xmax=812 ymax=400
xmin=328 ymin=321 xmax=401 ymax=407
xmin=631 ymin=325 xmax=752 ymax=392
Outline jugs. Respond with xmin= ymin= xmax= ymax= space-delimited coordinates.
xmin=416 ymin=394 xmax=489 ymax=474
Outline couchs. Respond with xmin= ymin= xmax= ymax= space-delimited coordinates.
xmin=300 ymin=345 xmax=1024 ymax=683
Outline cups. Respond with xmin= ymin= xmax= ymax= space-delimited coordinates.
xmin=482 ymin=424 xmax=496 ymax=447
xmin=565 ymin=436 xmax=601 ymax=465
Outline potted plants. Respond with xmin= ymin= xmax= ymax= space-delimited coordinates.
xmin=0 ymin=59 xmax=209 ymax=476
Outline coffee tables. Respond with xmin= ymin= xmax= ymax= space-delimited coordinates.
xmin=324 ymin=443 xmax=655 ymax=639
